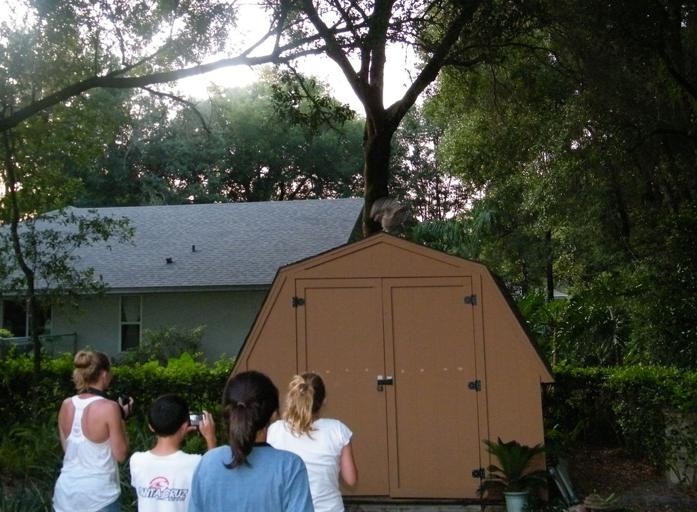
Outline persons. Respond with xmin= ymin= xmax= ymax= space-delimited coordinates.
xmin=129 ymin=394 xmax=218 ymax=512
xmin=50 ymin=346 xmax=135 ymax=512
xmin=187 ymin=370 xmax=316 ymax=512
xmin=267 ymin=373 xmax=358 ymax=512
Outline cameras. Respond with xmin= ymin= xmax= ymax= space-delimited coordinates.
xmin=189 ymin=414 xmax=203 ymax=426
xmin=108 ymin=390 xmax=129 ymax=409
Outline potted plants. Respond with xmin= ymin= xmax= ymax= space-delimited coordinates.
xmin=472 ymin=436 xmax=555 ymax=512
